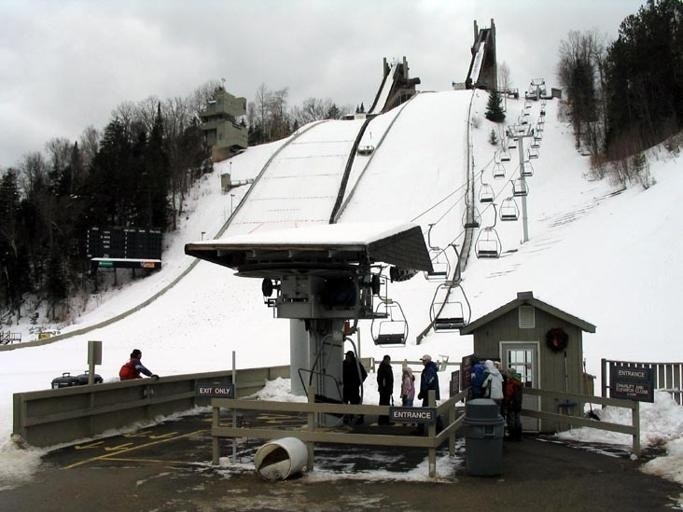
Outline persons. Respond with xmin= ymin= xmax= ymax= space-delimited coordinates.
xmin=414 ymin=354 xmax=444 ymax=435
xmin=129 ymin=350 xmax=159 ymax=378
xmin=377 ymin=355 xmax=395 ymax=426
xmin=342 ymin=350 xmax=368 ymax=424
xmin=399 ymin=366 xmax=415 ymax=426
xmin=469 ymin=358 xmax=522 ymax=442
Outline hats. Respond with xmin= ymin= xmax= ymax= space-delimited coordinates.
xmin=419 ymin=354 xmax=432 ymax=362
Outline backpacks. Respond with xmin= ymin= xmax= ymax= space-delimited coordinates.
xmin=119 ymin=360 xmax=139 ymax=380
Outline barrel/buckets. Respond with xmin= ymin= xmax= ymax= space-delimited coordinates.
xmin=255 ymin=435 xmax=309 ymax=481
xmin=461 ymin=414 xmax=505 ymax=477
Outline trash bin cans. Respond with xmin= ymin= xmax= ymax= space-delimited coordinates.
xmin=461 ymin=398 xmax=505 ymax=477
